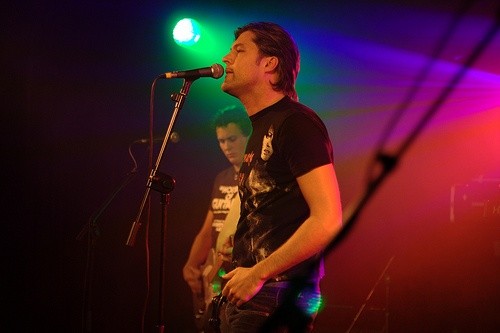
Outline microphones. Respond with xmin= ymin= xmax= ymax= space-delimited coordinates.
xmin=136 ymin=132 xmax=180 ymax=143
xmin=160 ymin=64 xmax=224 ymax=79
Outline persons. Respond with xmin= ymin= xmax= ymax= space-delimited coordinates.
xmin=200 ymin=20 xmax=343 ymax=333
xmin=183 ymin=107 xmax=253 ymax=311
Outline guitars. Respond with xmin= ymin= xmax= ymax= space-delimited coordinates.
xmin=192 ymin=234 xmax=235 ymax=330
